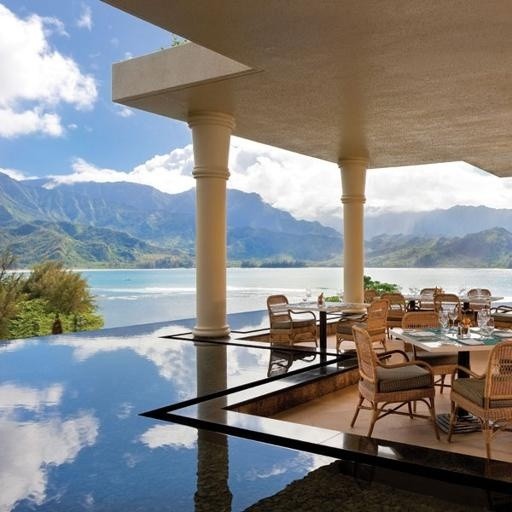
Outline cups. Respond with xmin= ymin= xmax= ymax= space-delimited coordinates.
xmin=408 ymin=287 xmax=420 ymax=300
xmin=318 ymin=296 xmax=325 ymax=308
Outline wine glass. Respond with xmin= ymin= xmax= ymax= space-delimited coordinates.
xmin=438 ymin=307 xmax=495 ymax=340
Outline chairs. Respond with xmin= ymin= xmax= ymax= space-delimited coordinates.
xmin=267 ymin=348 xmax=317 ymax=378
xmin=336 ymin=298 xmax=390 ymax=354
xmin=351 ymin=286 xmax=512 ymax=394
xmin=446 ymin=342 xmax=512 ymax=464
xmin=267 ymin=295 xmax=318 ymax=347
xmin=350 ymin=324 xmax=440 ymax=439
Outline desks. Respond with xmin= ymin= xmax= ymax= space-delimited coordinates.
xmin=278 ymin=301 xmax=371 ymax=356
xmin=388 ymin=325 xmax=511 ymax=434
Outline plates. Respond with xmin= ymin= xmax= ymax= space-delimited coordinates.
xmin=493 ymin=331 xmax=511 ymax=338
xmin=409 ymin=331 xmax=435 ymax=340
